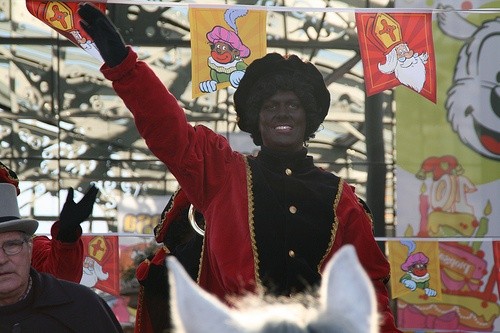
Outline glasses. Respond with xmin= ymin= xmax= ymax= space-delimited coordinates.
xmin=0 ymin=238 xmax=26 ymax=255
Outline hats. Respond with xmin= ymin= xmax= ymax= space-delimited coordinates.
xmin=233 ymin=52 xmax=330 ymax=135
xmin=0 ymin=161 xmax=20 ymax=197
xmin=153 ymin=186 xmax=190 ymax=242
xmin=0 ymin=182 xmax=39 ymax=235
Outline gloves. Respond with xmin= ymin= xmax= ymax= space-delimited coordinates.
xmin=56 ymin=184 xmax=99 ymax=242
xmin=77 ymin=3 xmax=127 ymax=68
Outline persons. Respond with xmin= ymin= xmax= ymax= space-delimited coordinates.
xmin=135 ymin=187 xmax=204 ymax=333
xmin=78 ymin=3 xmax=395 ymax=333
xmin=0 ymin=162 xmax=99 ymax=283
xmin=0 ymin=184 xmax=123 ymax=333
